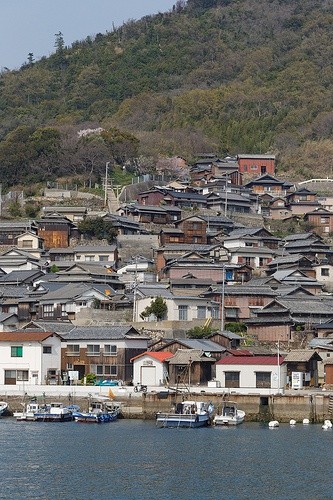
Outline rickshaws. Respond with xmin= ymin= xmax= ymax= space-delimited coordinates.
xmin=133 ymin=385 xmax=147 ymax=393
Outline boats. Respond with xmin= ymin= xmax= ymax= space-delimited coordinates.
xmin=71 ymin=399 xmax=122 ymax=424
xmin=0 ymin=401 xmax=8 ymax=416
xmin=11 ymin=401 xmax=74 ymax=423
xmin=154 ymin=400 xmax=214 ymax=430
xmin=211 ymin=404 xmax=246 ymax=427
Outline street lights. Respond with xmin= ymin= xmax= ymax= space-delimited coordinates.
xmin=104 ymin=160 xmax=110 ymax=207
xmin=188 ymin=356 xmax=193 ymax=392
xmin=281 ymin=241 xmax=289 ymax=258
